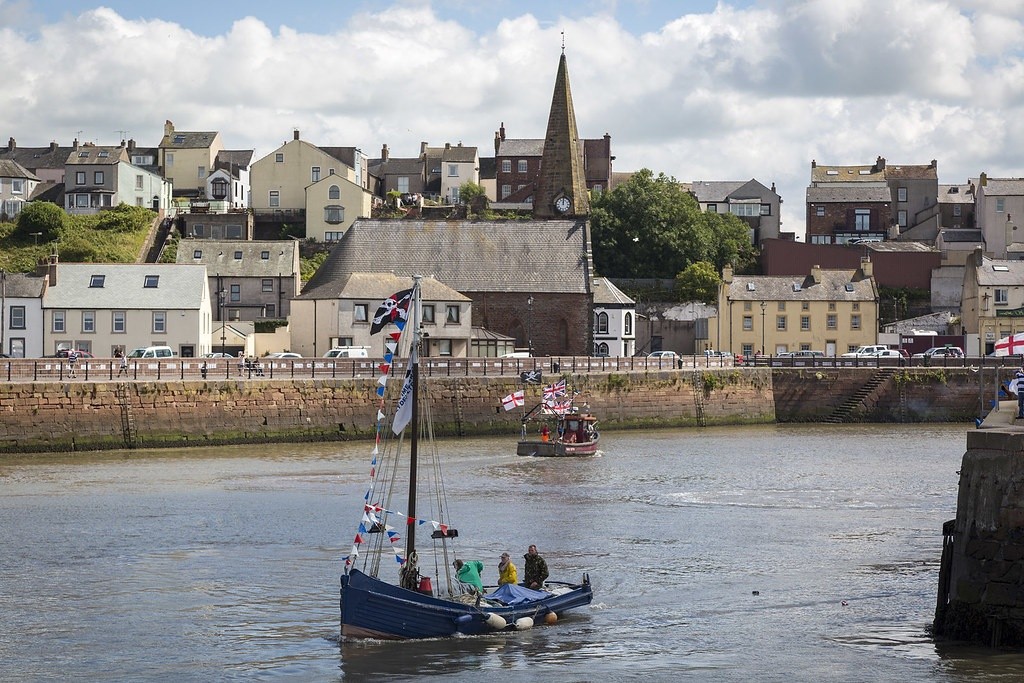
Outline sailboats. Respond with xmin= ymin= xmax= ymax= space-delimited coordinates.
xmin=338 ymin=274 xmax=594 ymax=641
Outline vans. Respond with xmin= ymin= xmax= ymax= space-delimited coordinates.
xmin=124 ymin=346 xmax=174 ymax=370
xmin=493 ymin=352 xmax=533 ymax=368
xmin=318 ymin=346 xmax=373 ymax=369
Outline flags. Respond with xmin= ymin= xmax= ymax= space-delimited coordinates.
xmin=501 ymin=390 xmax=524 ymax=411
xmin=370 ymin=289 xmax=412 ymax=335
xmin=542 ymin=400 xmax=571 ymax=415
xmin=391 ymin=354 xmax=412 ymax=435
xmin=520 ymin=369 xmax=542 ymax=383
xmin=994 ymin=333 xmax=1024 ymax=357
xmin=543 ymin=379 xmax=565 ymax=399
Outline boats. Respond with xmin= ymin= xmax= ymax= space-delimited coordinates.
xmin=515 ymin=385 xmax=602 ymax=457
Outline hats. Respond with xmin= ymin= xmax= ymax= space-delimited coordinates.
xmin=499 ymin=552 xmax=510 ymax=558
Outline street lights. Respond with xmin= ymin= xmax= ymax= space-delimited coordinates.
xmin=527 ymin=294 xmax=534 ymax=357
xmin=219 ymin=287 xmax=228 ymax=357
xmin=760 ymin=301 xmax=768 ymax=358
xmin=29 ymin=232 xmax=43 ymax=272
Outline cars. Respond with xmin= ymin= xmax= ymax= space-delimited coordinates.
xmin=196 ymin=353 xmax=234 ymax=369
xmin=776 ymin=349 xmax=825 ymax=358
xmin=259 ymin=353 xmax=311 ymax=369
xmin=0 ymin=352 xmax=15 ymax=359
xmin=648 ymin=351 xmax=683 ymax=357
xmin=862 ymin=350 xmax=903 ymax=358
xmin=54 ymin=351 xmax=95 ymax=358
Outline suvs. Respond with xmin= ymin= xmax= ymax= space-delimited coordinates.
xmin=841 ymin=345 xmax=887 ymax=357
xmin=912 ymin=346 xmax=965 ymax=358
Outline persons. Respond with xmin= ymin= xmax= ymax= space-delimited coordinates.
xmin=498 ymin=552 xmax=517 ymax=585
xmin=541 ymin=425 xmax=551 ymax=440
xmin=558 ymin=423 xmax=564 ymax=436
xmin=118 ymin=351 xmax=128 ymax=378
xmin=523 ymin=545 xmax=549 ymax=590
xmin=237 ymin=354 xmax=264 ymax=377
xmin=1015 ymin=371 xmax=1024 ymax=418
xmin=756 ymin=349 xmax=762 ymax=355
xmin=453 ymin=559 xmax=483 ymax=593
xmin=945 ymin=347 xmax=950 ymax=353
xmin=67 ymin=349 xmax=78 ymax=378
